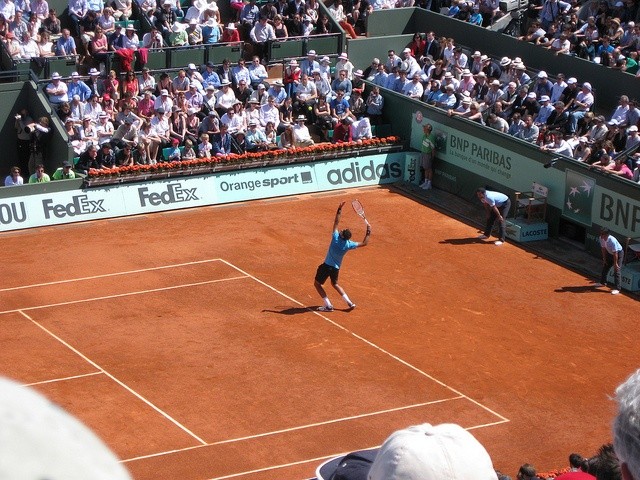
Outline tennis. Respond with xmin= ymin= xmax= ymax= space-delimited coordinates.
xmin=308 ymin=106 xmax=312 ymax=110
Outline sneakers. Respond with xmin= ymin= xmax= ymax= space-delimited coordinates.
xmin=319 ymin=306 xmax=334 ymax=311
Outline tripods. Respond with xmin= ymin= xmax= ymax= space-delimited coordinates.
xmin=23 ymin=133 xmax=46 ymax=174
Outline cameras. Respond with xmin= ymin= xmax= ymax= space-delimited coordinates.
xmin=155 ymin=36 xmax=160 ymax=43
xmin=14 ymin=114 xmax=24 ymax=121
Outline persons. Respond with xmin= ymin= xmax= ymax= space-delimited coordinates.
xmin=476 ymin=187 xmax=511 ymax=246
xmin=568 ymin=453 xmax=582 ymax=471
xmin=52 ymin=161 xmax=76 ymax=180
xmin=385 ymin=0 xmax=487 ymax=126
xmin=487 ymin=0 xmax=640 ymax=185
xmin=5 ymin=167 xmax=23 ymax=186
xmin=610 ymin=366 xmax=639 ymax=480
xmin=597 ymin=444 xmax=621 ymax=480
xmin=1 ymin=0 xmax=384 ymax=59
xmin=417 ymin=124 xmax=437 ymax=190
xmin=313 ymin=199 xmax=372 ymax=313
xmin=29 ymin=164 xmax=50 ymax=184
xmin=46 ymin=50 xmax=385 ymax=174
xmin=592 ymin=226 xmax=624 ymax=295
xmin=14 ymin=107 xmax=52 ymax=174
xmin=517 ymin=463 xmax=536 ymax=480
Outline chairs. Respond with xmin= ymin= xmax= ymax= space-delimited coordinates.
xmin=264 ymin=63 xmax=284 ymax=86
xmin=513 ymin=181 xmax=550 ymax=224
xmin=625 ymin=235 xmax=640 ymax=270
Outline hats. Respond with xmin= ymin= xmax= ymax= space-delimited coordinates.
xmin=567 ymin=77 xmax=577 ymax=84
xmin=538 ymin=71 xmax=548 ymax=78
xmin=460 ymin=97 xmax=472 ymax=104
xmin=187 ymin=108 xmax=200 ymax=116
xmin=479 ymin=54 xmax=492 ymax=63
xmin=500 ymin=56 xmax=511 ymax=66
xmin=234 ymin=130 xmax=245 ymax=136
xmin=490 ymin=79 xmax=503 ymax=87
xmin=69 ymin=71 xmax=82 ymax=78
xmin=320 ymin=57 xmax=331 ymax=63
xmin=249 ymin=120 xmax=256 ymax=126
xmin=248 ymin=97 xmax=260 ymax=104
xmin=89 ymin=5 xmax=100 ymax=11
xmin=63 ymin=161 xmax=71 ymax=167
xmin=616 ymin=2 xmax=624 ymax=7
xmin=102 ymin=142 xmax=112 ymax=149
xmin=205 ymin=86 xmax=215 ymax=91
xmin=103 ymin=94 xmax=110 ymax=101
xmin=422 ymin=124 xmax=432 ymax=131
xmin=346 ymin=116 xmax=353 ymax=124
xmin=444 ymin=72 xmax=455 ymax=79
xmin=273 ymin=79 xmax=285 ymax=86
xmin=201 ymin=134 xmax=209 ymax=140
xmin=608 ymin=119 xmax=618 ymax=125
xmin=171 ymin=21 xmax=183 ymax=33
xmin=471 ymin=51 xmax=481 ymax=59
xmin=189 ymin=18 xmax=197 ymax=24
xmin=579 ymin=136 xmax=588 ymax=142
xmin=594 ymin=115 xmax=605 ymax=122
xmin=88 ymin=68 xmax=100 ymax=76
xmin=473 ymin=72 xmax=487 ymax=79
xmin=307 ymin=49 xmax=318 ymax=57
xmin=232 ymin=99 xmax=242 ymax=106
xmin=462 ymin=69 xmax=473 ymax=77
xmin=611 ymin=18 xmax=620 ymax=26
xmin=512 ymin=56 xmax=523 ymax=65
xmin=258 ymin=84 xmax=266 ymax=90
xmin=460 ymin=90 xmax=470 ymax=98
xmin=295 ymin=114 xmax=308 ymax=121
xmin=146 ymin=7 xmax=156 ymax=15
xmin=403 ymin=48 xmax=411 ymax=52
xmin=206 ymin=62 xmax=216 ymax=68
xmin=208 ymin=111 xmax=215 ymax=117
xmin=337 ymin=53 xmax=349 ymax=60
xmin=124 ymin=118 xmax=133 ymax=124
xmin=423 ymin=55 xmax=435 ymax=64
xmin=225 ymin=23 xmax=237 ymax=30
xmin=52 ymin=71 xmax=62 ymax=80
xmin=315 ymin=448 xmax=380 ymax=480
xmin=207 ymin=1 xmax=218 ymax=11
xmin=83 ymin=115 xmax=91 ymax=122
xmin=311 ymin=68 xmax=321 ymax=74
xmin=188 ymin=63 xmax=197 ymax=69
xmin=161 ymin=89 xmax=169 ymax=96
xmin=189 ymin=82 xmax=199 ymax=89
xmin=445 ymin=84 xmax=456 ymax=92
xmin=288 ymin=60 xmax=299 ymax=66
xmin=260 ymin=15 xmax=267 ymax=20
xmin=369 ymin=423 xmax=497 ymax=480
xmin=354 ymin=70 xmax=365 ymax=76
xmin=157 ymin=108 xmax=164 ymax=113
xmin=172 ymin=139 xmax=179 ymax=144
xmin=126 ymin=24 xmax=136 ymax=31
xmin=205 ymin=18 xmax=217 ymax=27
xmin=304 ymin=16 xmax=312 ymax=22
xmin=355 ymin=89 xmax=361 ymax=94
xmin=539 ymin=95 xmax=550 ymax=102
xmin=528 ymin=92 xmax=536 ymax=99
xmin=473 ymin=5 xmax=479 ymax=9
xmin=220 ymin=79 xmax=232 ymax=87
xmin=171 ymin=105 xmax=182 ymax=113
xmin=6 ymin=33 xmax=13 ymax=39
xmin=162 ymin=0 xmax=175 ymax=9
xmin=400 ymin=61 xmax=411 ymax=73
xmin=39 ymin=117 xmax=49 ymax=126
xmin=627 ymin=125 xmax=638 ymax=133
xmin=99 ymin=111 xmax=108 ymax=119
xmin=194 ymin=0 xmax=207 ymax=11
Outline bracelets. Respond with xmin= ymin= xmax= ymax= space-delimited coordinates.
xmin=366 ymin=230 xmax=370 ymax=236
xmin=337 ymin=208 xmax=342 ymax=215
